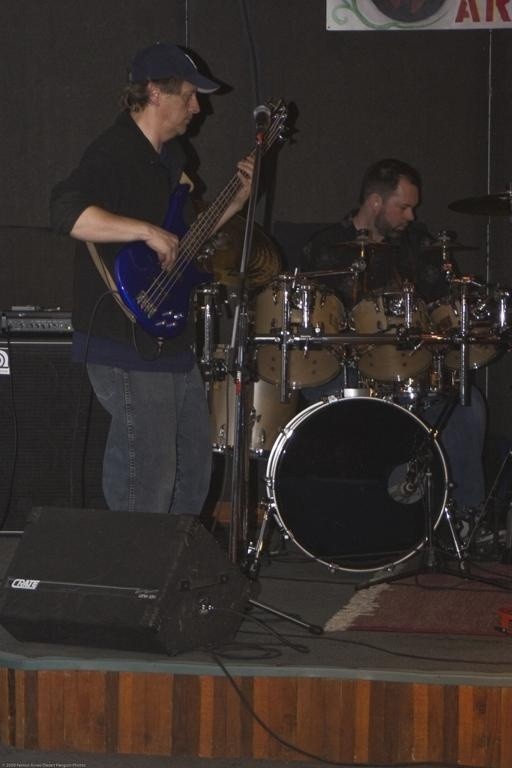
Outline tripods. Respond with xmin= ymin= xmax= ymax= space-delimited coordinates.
xmin=230 ymin=141 xmax=323 ymax=635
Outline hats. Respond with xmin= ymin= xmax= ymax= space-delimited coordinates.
xmin=131 ymin=43 xmax=220 ymax=94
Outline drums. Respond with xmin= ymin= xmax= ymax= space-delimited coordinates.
xmin=202 ymin=345 xmax=300 ymax=461
xmin=265 ymin=394 xmax=449 ymax=573
xmin=248 ymin=273 xmax=348 ymax=389
xmin=427 ymin=295 xmax=508 ymax=371
xmin=348 ymin=287 xmax=439 ymax=385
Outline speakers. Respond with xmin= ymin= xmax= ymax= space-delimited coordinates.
xmin=0 ymin=505 xmax=261 ymax=654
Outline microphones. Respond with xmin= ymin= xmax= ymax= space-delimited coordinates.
xmin=253 ymin=105 xmax=271 ymax=141
xmin=0 ymin=338 xmax=113 ymax=538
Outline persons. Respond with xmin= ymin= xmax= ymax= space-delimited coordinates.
xmin=49 ymin=44 xmax=256 ymax=515
xmin=299 ymin=157 xmax=488 ymax=513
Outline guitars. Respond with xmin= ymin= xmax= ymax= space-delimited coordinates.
xmin=113 ymin=96 xmax=290 ymax=341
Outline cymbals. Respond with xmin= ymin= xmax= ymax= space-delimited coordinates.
xmin=447 ymin=192 xmax=511 ymax=216
xmin=300 ymin=270 xmax=359 ymax=278
xmin=420 ymin=245 xmax=481 ymax=251
xmin=330 ymin=239 xmax=399 ymax=251
xmin=194 ymin=199 xmax=283 ymax=292
